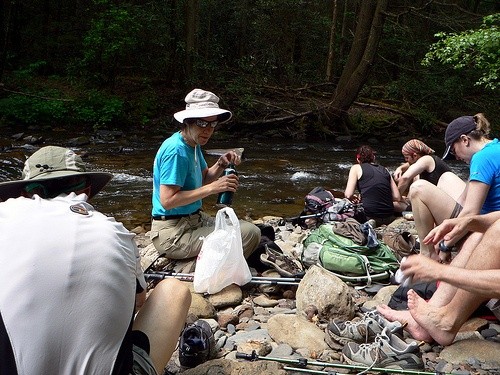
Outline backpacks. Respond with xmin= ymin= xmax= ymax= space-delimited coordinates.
xmin=304 ymin=186 xmax=335 ymax=214
xmin=301 ymin=221 xmax=401 ymax=282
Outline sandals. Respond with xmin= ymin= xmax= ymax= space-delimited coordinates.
xmin=260 ymin=244 xmax=306 ymax=278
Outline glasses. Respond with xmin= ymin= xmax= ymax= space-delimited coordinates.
xmin=194 ymin=118 xmax=219 ymax=128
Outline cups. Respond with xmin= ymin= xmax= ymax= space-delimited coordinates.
xmin=217 ymin=161 xmax=237 ymax=206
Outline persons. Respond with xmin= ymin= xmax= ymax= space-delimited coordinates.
xmin=377 ymin=210 xmax=500 ymax=346
xmin=0 ymin=146 xmax=192 ymax=375
xmin=394 ymin=139 xmax=452 ymax=197
xmin=409 ymin=114 xmax=500 ymax=263
xmin=393 ymin=201 xmax=414 ymax=215
xmin=344 ymin=146 xmax=410 ymax=227
xmin=145 ymin=89 xmax=261 ymax=261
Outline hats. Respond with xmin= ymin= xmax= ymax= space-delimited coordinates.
xmin=402 ymin=139 xmax=435 ymax=158
xmin=0 ymin=146 xmax=114 ymax=202
xmin=441 ymin=116 xmax=476 ymax=161
xmin=174 ymin=89 xmax=232 ymax=124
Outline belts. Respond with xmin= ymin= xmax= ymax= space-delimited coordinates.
xmin=152 ymin=209 xmax=199 ymax=220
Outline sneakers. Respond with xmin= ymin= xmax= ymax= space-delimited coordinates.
xmin=382 ymin=231 xmax=416 ymax=265
xmin=325 ymin=311 xmax=408 ymax=352
xmin=179 ymin=319 xmax=215 ymax=367
xmin=342 ymin=325 xmax=425 ymax=373
